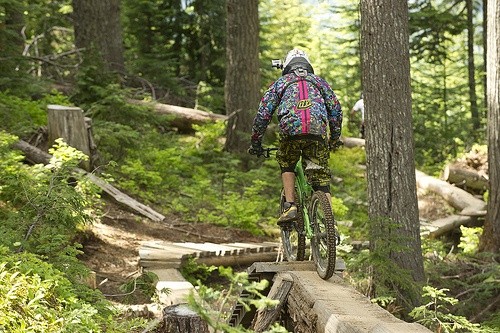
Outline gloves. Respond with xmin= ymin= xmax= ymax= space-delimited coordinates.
xmin=248 ymin=144 xmax=263 ymax=159
xmin=328 ymin=136 xmax=344 ymax=151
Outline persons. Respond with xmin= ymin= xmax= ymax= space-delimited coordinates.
xmin=350 ymin=93 xmax=365 ymax=140
xmin=247 ymin=49 xmax=343 ymax=247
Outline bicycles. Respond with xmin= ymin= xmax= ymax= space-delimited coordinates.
xmin=249 ymin=142 xmax=344 ymax=280
xmin=349 ymin=120 xmax=365 ymax=139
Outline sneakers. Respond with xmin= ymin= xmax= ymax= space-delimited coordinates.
xmin=277 ymin=204 xmax=298 ymax=225
xmin=321 ymin=218 xmax=338 ymax=233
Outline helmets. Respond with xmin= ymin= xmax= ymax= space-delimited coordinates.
xmin=280 ymin=49 xmax=314 ymax=75
